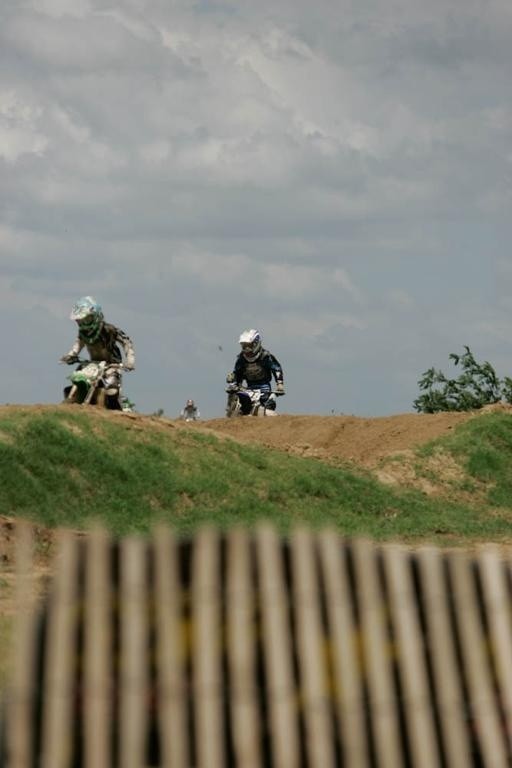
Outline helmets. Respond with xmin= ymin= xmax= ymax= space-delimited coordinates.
xmin=186 ymin=399 xmax=195 ymax=411
xmin=120 ymin=396 xmax=130 ymax=407
xmin=240 ymin=329 xmax=261 ymax=358
xmin=71 ymin=296 xmax=104 ymax=343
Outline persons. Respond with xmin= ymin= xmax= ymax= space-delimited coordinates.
xmin=182 ymin=399 xmax=200 ymax=420
xmin=61 ymin=297 xmax=135 ymax=410
xmin=225 ymin=329 xmax=283 ymax=417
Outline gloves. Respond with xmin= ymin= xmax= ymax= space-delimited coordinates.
xmin=275 ymin=386 xmax=284 ymax=397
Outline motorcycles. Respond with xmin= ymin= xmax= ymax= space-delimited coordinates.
xmin=224 ymin=377 xmax=286 ymax=416
xmin=58 ymin=353 xmax=134 ymax=405
